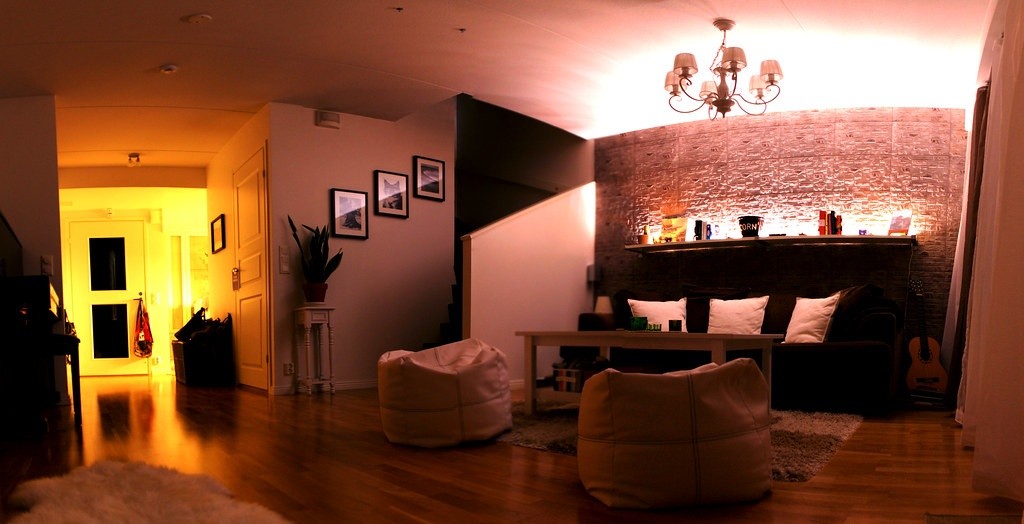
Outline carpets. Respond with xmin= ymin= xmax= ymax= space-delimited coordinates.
xmin=0 ymin=454 xmax=292 ymax=524
xmin=496 ymin=396 xmax=863 ymax=484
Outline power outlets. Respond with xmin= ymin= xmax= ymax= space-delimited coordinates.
xmin=284 ymin=363 xmax=294 ymax=375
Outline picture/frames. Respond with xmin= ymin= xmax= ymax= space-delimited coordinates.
xmin=330 ymin=188 xmax=369 ymax=240
xmin=210 ymin=213 xmax=226 ymax=254
xmin=412 ymin=155 xmax=446 ymax=202
xmin=373 ymin=169 xmax=409 ymax=219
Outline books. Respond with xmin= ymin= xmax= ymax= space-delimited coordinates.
xmin=818 ymin=210 xmax=842 ymax=235
xmin=695 ymin=220 xmax=711 ymax=240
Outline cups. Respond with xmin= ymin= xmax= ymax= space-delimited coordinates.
xmin=630 ymin=317 xmax=647 ymax=330
xmin=669 ymin=320 xmax=682 ymax=331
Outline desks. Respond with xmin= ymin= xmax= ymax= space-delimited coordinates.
xmin=293 ymin=306 xmax=337 ymax=397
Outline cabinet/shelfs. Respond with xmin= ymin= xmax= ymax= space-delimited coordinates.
xmin=0 ymin=273 xmax=61 ymax=445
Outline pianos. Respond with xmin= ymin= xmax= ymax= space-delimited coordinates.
xmin=0 ymin=275 xmax=60 ymax=443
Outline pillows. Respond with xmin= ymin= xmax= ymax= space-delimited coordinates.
xmin=626 ymin=295 xmax=688 ymax=334
xmin=706 ymin=295 xmax=770 ymax=335
xmin=780 ymin=291 xmax=841 ymax=344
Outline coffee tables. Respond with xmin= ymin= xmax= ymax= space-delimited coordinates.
xmin=515 ymin=329 xmax=784 ymax=417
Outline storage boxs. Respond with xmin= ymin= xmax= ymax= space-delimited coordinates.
xmin=553 ymin=367 xmax=582 ymax=392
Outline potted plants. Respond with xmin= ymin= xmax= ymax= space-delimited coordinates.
xmin=287 ymin=213 xmax=343 ymax=302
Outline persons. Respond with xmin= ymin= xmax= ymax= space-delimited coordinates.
xmin=891 ymin=216 xmax=911 ymax=230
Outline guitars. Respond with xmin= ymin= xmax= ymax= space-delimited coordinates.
xmin=905 ymin=278 xmax=949 ymax=395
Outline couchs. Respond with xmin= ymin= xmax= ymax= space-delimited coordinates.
xmin=552 ymin=283 xmax=904 ymax=419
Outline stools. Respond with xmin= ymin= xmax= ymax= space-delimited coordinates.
xmin=377 ymin=337 xmax=514 ymax=448
xmin=43 ymin=333 xmax=81 ymax=424
xmin=575 ymin=358 xmax=775 ymax=511
xmin=173 ymin=333 xmax=232 ymax=386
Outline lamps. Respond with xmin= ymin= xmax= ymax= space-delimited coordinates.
xmin=128 ymin=153 xmax=141 ymax=167
xmin=664 ymin=19 xmax=783 ymax=122
